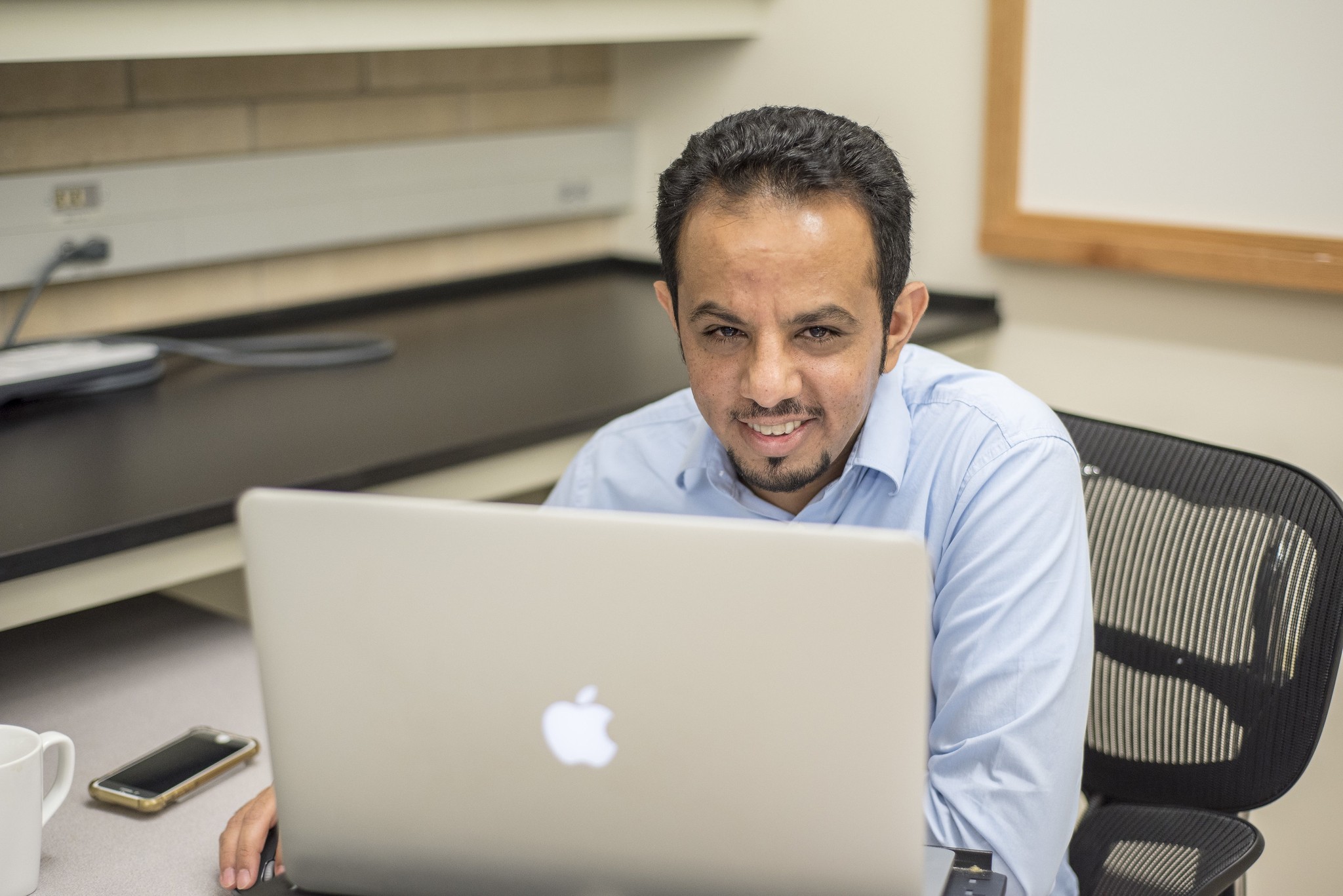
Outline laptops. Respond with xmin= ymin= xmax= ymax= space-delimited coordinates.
xmin=235 ymin=486 xmax=955 ymax=896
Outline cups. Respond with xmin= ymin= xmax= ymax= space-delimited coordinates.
xmin=0 ymin=724 xmax=75 ymax=896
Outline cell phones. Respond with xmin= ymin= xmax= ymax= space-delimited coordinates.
xmin=89 ymin=726 xmax=258 ymax=813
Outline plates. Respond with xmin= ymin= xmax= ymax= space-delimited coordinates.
xmin=1 ymin=339 xmax=158 ymax=397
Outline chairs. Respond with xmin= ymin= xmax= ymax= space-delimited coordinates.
xmin=1052 ymin=411 xmax=1343 ymax=896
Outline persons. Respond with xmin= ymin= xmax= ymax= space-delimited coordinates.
xmin=218 ymin=105 xmax=1093 ymax=896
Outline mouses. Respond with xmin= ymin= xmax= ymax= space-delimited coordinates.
xmin=237 ymin=823 xmax=297 ymax=896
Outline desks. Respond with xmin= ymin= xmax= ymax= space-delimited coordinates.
xmin=0 ymin=257 xmax=998 ymax=636
xmin=0 ymin=593 xmax=272 ymax=896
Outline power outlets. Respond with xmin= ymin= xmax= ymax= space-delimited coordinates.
xmin=54 ymin=214 xmax=109 ymax=268
